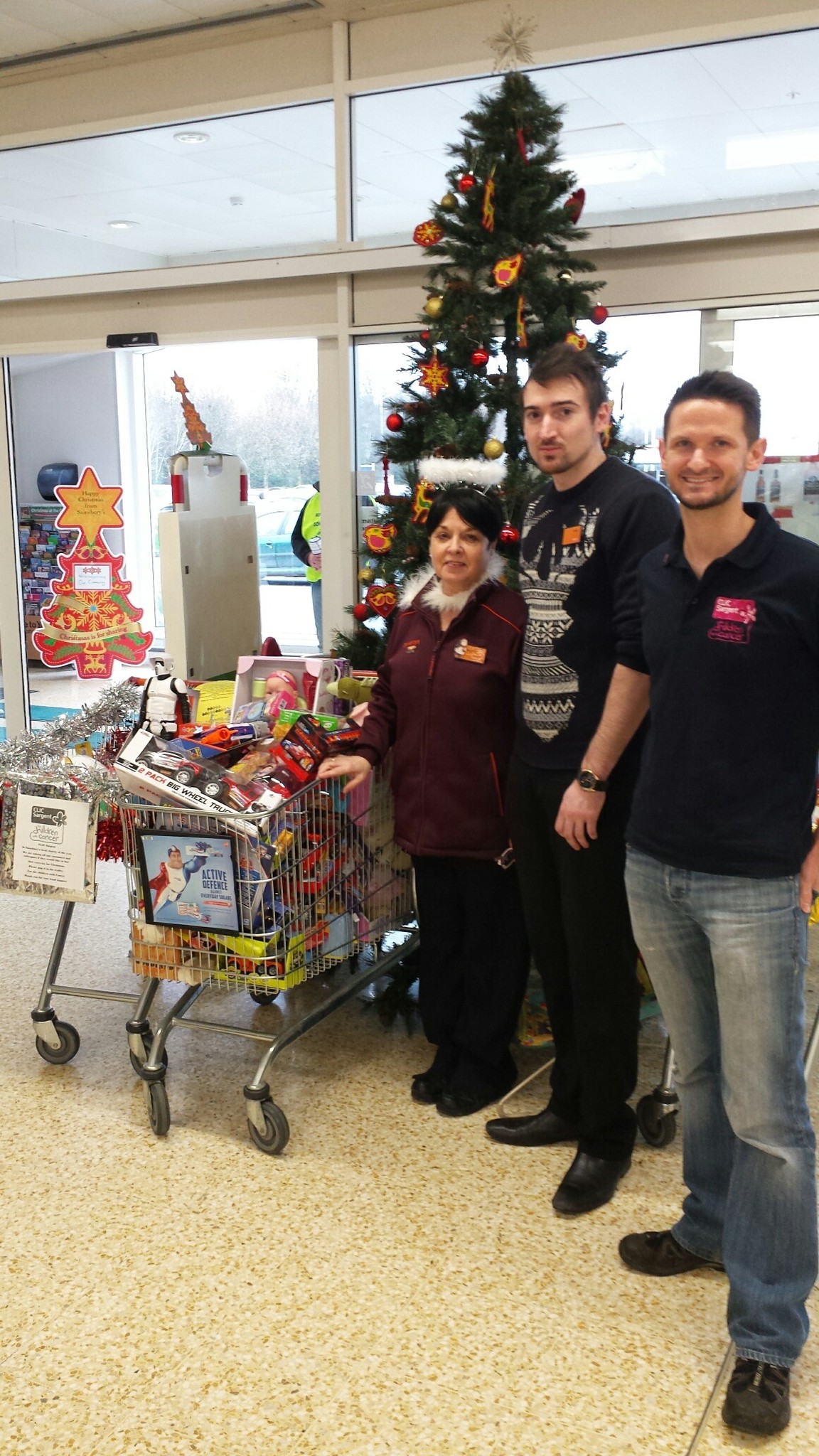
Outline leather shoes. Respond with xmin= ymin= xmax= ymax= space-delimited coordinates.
xmin=436 ymin=1066 xmax=519 ymax=1116
xmin=485 ymin=1108 xmax=579 ymax=1147
xmin=411 ymin=1062 xmax=453 ymax=1105
xmin=551 ymin=1144 xmax=632 ymax=1214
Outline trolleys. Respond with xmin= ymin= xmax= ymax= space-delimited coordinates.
xmin=1 ymin=679 xmax=221 ymax=1067
xmin=113 ymin=779 xmax=423 ymax=1154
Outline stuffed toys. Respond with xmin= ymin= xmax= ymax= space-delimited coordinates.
xmin=325 ymin=676 xmax=379 ymax=705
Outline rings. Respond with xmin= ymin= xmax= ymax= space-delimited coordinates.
xmin=331 ymin=758 xmax=335 ymax=762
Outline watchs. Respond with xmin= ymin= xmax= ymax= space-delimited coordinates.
xmin=574 ymin=763 xmax=607 ymax=793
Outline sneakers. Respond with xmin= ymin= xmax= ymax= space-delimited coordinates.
xmin=618 ymin=1230 xmax=726 ymax=1277
xmin=721 ymin=1357 xmax=791 ymax=1436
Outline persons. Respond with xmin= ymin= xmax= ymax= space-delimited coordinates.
xmin=291 ymin=479 xmax=380 ymax=654
xmin=483 ymin=341 xmax=680 ymax=1219
xmin=313 ymin=486 xmax=535 ymax=1120
xmin=263 ymin=670 xmax=298 ymax=707
xmin=614 ymin=371 xmax=819 ymax=1435
xmin=131 ymin=653 xmax=190 ymax=740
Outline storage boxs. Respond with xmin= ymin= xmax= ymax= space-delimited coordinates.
xmin=112 ymin=655 xmax=350 ymax=843
